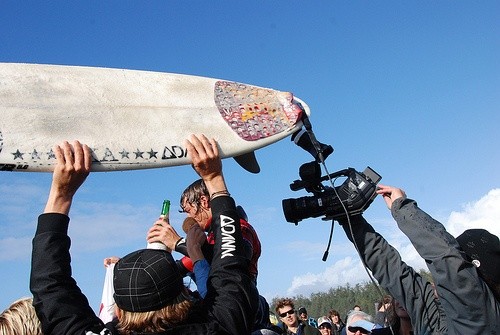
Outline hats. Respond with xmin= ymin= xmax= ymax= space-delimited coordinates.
xmin=456 ymin=228 xmax=500 ymax=285
xmin=113 ymin=249 xmax=185 ymax=313
xmin=317 ymin=316 xmax=332 ymax=327
xmin=297 ymin=306 xmax=306 ymax=311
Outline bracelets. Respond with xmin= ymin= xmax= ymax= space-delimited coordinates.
xmin=210 ymin=190 xmax=230 ymax=201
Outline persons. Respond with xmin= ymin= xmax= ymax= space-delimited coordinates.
xmin=254 ymin=295 xmax=414 ymax=335
xmin=30 ymin=132 xmax=259 ymax=335
xmin=0 ymin=297 xmax=44 ymax=335
xmin=104 ymin=179 xmax=262 ymax=288
xmin=336 ymin=185 xmax=500 ymax=335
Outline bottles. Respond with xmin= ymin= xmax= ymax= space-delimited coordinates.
xmin=153 ymin=200 xmax=171 ymax=243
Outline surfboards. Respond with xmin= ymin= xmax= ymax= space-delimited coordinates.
xmin=0 ymin=62 xmax=310 ymax=174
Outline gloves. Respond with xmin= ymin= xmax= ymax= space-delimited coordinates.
xmin=349 ymin=320 xmax=375 ymax=332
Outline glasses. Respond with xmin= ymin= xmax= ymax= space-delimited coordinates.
xmin=348 ymin=327 xmax=370 ymax=334
xmin=318 ymin=324 xmax=332 ymax=330
xmin=279 ymin=309 xmax=295 ymax=317
